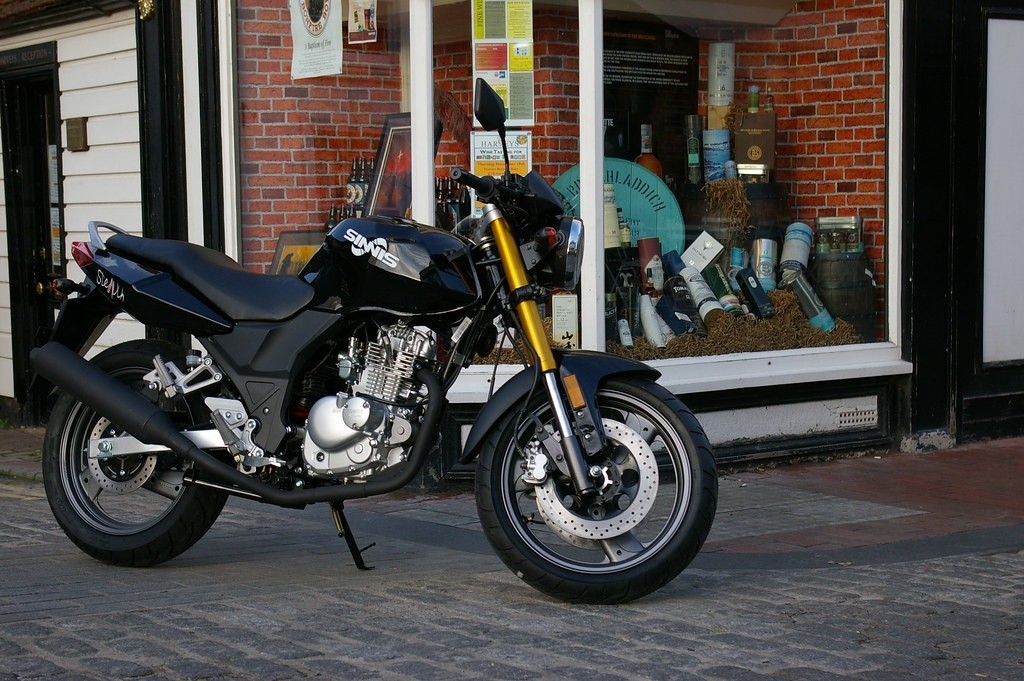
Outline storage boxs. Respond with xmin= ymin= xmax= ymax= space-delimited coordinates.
xmin=734 ymin=112 xmax=776 ymax=169
xmin=552 ymin=295 xmax=578 ymax=350
xmin=680 ymin=231 xmax=724 ymax=273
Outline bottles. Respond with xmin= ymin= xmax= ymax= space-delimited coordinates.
xmin=435 ymin=176 xmax=470 ymax=231
xmin=634 ymin=124 xmax=661 ymax=179
xmin=604 ymin=184 xmax=657 ymax=341
xmin=816 ymin=217 xmax=859 ymax=252
xmin=324 ymin=157 xmax=374 ymax=231
xmin=764 ymin=87 xmax=773 ymax=111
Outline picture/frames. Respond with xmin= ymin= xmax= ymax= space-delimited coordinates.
xmin=269 ymin=229 xmax=329 ymax=275
xmin=361 ymin=112 xmax=444 ymax=219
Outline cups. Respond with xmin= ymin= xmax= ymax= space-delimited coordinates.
xmin=686 ymin=115 xmax=701 ymax=183
xmin=748 ymin=86 xmax=759 ymax=113
xmin=779 ymin=272 xmax=836 ymax=334
xmin=705 ymin=265 xmax=745 ymax=317
xmin=680 ymin=267 xmax=725 ymax=327
xmin=736 ymin=268 xmax=775 ymax=318
xmin=364 ymin=9 xmax=370 ymax=30
xmin=780 ymin=222 xmax=813 ymax=272
xmin=663 ymin=275 xmax=708 ymax=340
xmin=724 ymin=161 xmax=737 ymax=181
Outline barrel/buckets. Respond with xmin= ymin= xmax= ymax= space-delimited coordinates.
xmin=808 ymin=251 xmax=873 ymax=337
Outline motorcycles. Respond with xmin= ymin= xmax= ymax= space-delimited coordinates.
xmin=29 ymin=78 xmax=719 ymax=607
